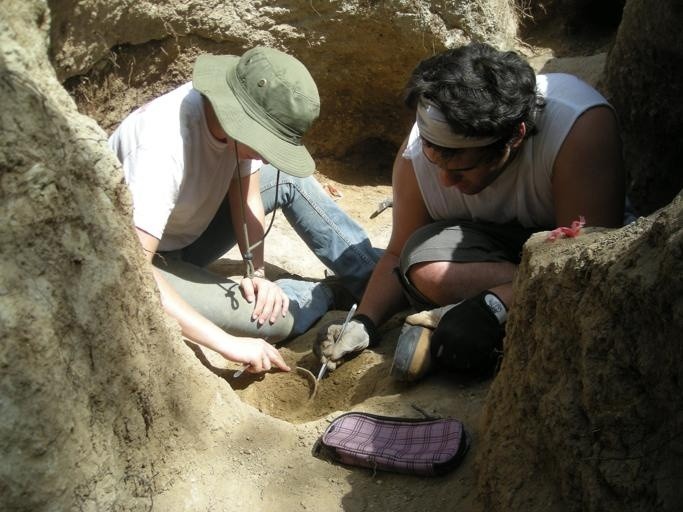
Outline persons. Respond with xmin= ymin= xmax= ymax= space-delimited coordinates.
xmin=318 ymin=40 xmax=627 ymax=390
xmin=106 ymin=44 xmax=382 ymax=378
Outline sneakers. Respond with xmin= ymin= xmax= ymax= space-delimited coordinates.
xmin=271 ymin=273 xmax=359 ymax=312
xmin=390 ymin=321 xmax=434 ymax=382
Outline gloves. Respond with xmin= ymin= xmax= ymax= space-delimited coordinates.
xmin=313 ymin=314 xmax=375 ymax=371
xmin=406 ymin=289 xmax=508 ymax=382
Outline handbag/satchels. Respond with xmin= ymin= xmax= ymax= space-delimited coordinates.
xmin=322 ymin=411 xmax=472 ymax=478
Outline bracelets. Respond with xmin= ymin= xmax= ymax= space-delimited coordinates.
xmin=349 ymin=313 xmax=381 ymax=350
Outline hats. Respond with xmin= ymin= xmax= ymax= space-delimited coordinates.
xmin=193 ymin=46 xmax=319 ymax=178
